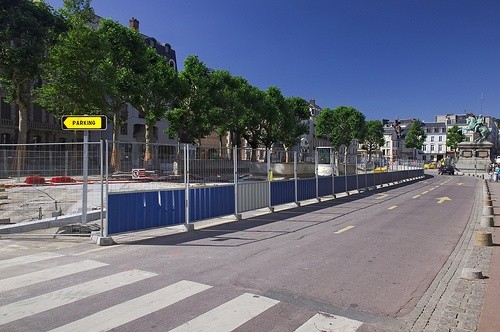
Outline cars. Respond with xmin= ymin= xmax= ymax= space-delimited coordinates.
xmin=494 ymin=155 xmax=500 ymax=164
xmin=438 ymin=165 xmax=454 ymax=175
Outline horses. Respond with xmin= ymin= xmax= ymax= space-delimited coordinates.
xmin=457 ymin=116 xmax=493 ymax=143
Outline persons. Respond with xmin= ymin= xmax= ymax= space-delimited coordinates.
xmin=473 ymin=113 xmax=486 ymax=134
xmin=436 ymin=155 xmax=456 ymax=169
xmin=484 ymin=159 xmax=500 ymax=182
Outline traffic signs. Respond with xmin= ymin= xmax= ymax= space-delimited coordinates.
xmin=61 ymin=114 xmax=108 ymax=131
